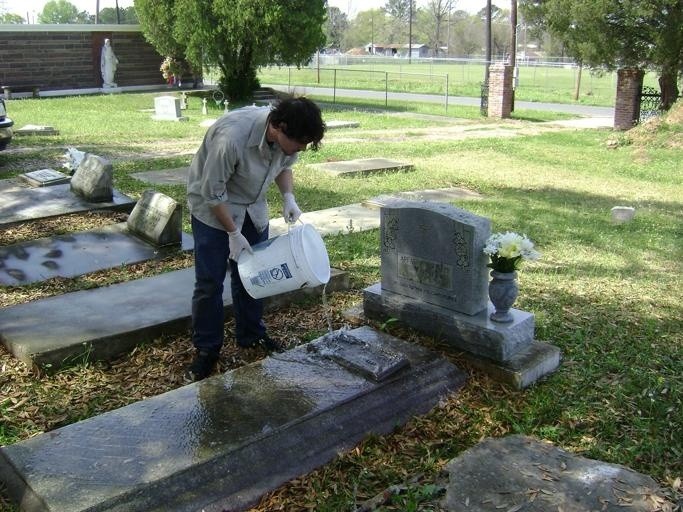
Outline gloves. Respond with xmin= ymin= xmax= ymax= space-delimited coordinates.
xmin=284 ymin=192 xmax=301 ymax=223
xmin=227 ymin=228 xmax=254 ymax=262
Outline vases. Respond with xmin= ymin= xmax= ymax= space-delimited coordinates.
xmin=488 ymin=269 xmax=519 ymax=323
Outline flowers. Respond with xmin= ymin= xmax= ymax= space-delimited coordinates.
xmin=483 ymin=231 xmax=542 ymax=274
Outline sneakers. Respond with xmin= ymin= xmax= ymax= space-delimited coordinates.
xmin=238 ymin=333 xmax=281 ymax=353
xmin=185 ymin=347 xmax=219 ymax=380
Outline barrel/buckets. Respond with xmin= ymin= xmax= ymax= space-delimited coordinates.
xmin=236 ymin=222 xmax=332 ymax=300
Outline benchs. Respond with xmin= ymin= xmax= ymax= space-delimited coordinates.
xmin=1 ymin=84 xmax=48 ymax=100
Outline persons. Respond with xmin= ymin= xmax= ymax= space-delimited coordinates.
xmin=182 ymin=95 xmax=326 ymax=381
xmin=100 ymin=38 xmax=119 ymax=85
